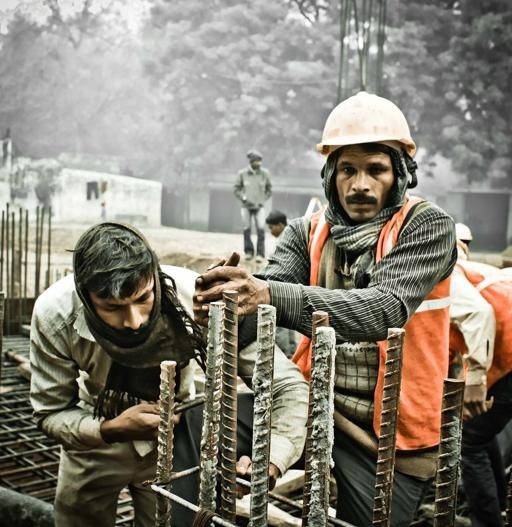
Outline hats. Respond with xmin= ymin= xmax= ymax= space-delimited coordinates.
xmin=246 ymin=149 xmax=263 ymax=160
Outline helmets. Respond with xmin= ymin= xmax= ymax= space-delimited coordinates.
xmin=455 ymin=223 xmax=472 ymax=241
xmin=321 ymin=91 xmax=410 ymax=146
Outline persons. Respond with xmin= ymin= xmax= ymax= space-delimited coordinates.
xmin=193 ymin=92 xmax=458 ymax=527
xmin=266 ymin=210 xmax=297 ymax=359
xmin=447 ymin=223 xmax=511 ymax=527
xmin=233 ymin=151 xmax=273 ymax=262
xmin=30 ymin=222 xmax=309 ymax=527
